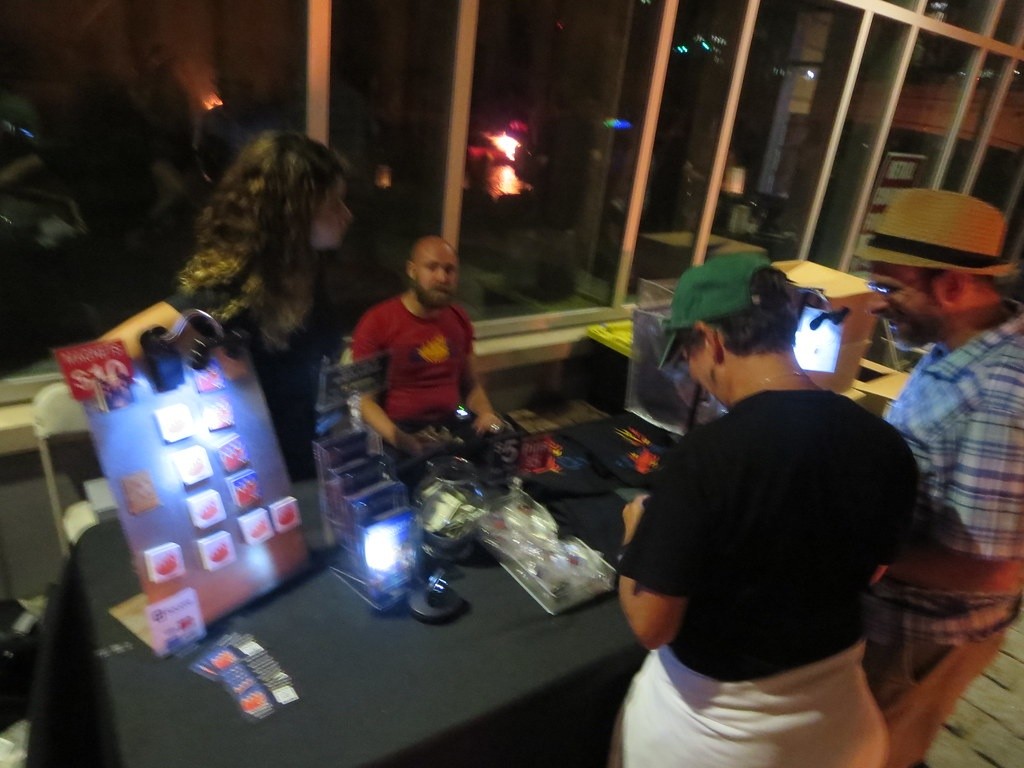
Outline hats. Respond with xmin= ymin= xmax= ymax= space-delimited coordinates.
xmin=852 ymin=188 xmax=1015 ymax=275
xmin=658 ymin=251 xmax=773 ymax=370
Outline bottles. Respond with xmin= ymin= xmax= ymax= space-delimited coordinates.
xmin=413 ymin=456 xmax=487 ymax=564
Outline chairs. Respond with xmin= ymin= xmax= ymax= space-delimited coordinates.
xmin=33 ymin=385 xmax=90 ymax=558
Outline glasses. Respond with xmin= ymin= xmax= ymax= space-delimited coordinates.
xmin=867 ymin=280 xmax=943 ymax=297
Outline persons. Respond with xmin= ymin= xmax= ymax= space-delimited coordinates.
xmin=610 ymin=189 xmax=1023 ymax=768
xmin=91 ymin=128 xmax=354 ymax=483
xmin=349 ymin=236 xmax=501 ymax=463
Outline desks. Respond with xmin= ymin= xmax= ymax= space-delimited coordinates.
xmin=56 ymin=410 xmax=671 ymax=768
